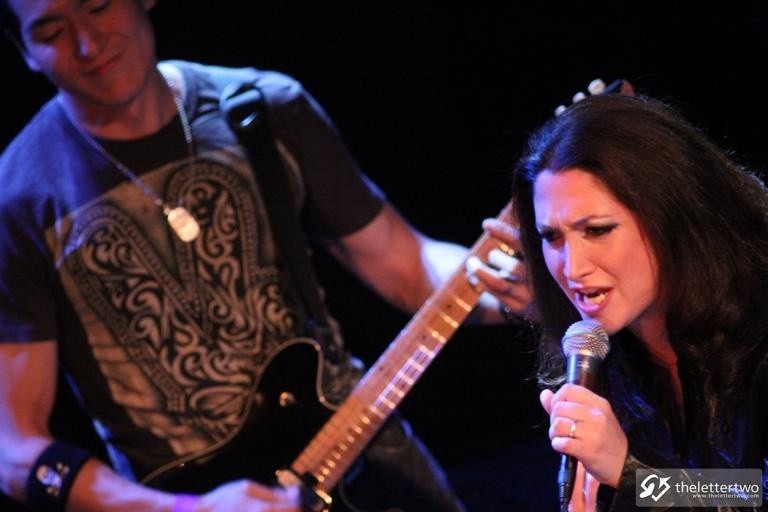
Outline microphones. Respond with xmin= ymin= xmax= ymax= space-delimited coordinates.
xmin=558 ymin=320 xmax=611 ymax=505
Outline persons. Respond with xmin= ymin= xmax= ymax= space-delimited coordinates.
xmin=0 ymin=1 xmax=541 ymax=512
xmin=510 ymin=89 xmax=767 ymax=512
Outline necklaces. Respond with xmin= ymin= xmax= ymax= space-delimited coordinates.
xmin=61 ymin=81 xmax=201 ymax=243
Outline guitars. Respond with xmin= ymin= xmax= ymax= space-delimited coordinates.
xmin=142 ymin=78 xmax=636 ymax=512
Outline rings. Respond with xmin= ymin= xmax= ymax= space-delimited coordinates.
xmin=568 ymin=420 xmax=577 ymax=437
